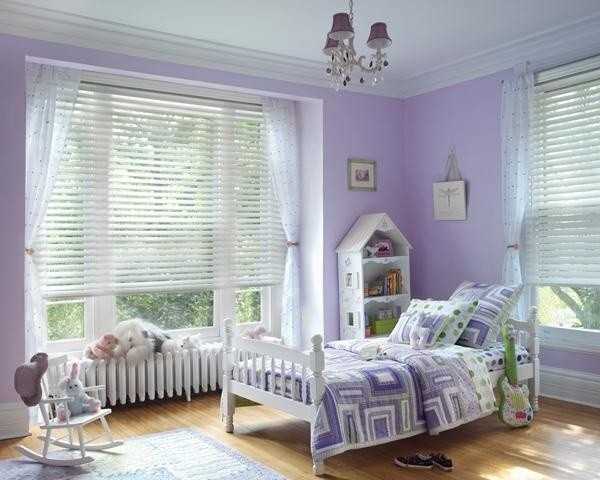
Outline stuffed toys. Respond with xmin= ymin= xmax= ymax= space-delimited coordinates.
xmin=243 ymin=326 xmax=266 ymax=339
xmin=57 ymin=362 xmax=101 ymax=420
xmin=84 ymin=318 xmax=202 ymax=360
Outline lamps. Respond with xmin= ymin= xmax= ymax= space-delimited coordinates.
xmin=323 ymin=0 xmax=392 ymax=93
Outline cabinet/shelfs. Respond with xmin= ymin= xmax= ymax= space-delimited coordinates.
xmin=334 ymin=212 xmax=414 ymax=342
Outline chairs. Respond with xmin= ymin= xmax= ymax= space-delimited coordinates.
xmin=14 ymin=353 xmax=123 ymax=468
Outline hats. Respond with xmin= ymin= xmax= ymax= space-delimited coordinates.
xmin=14 ymin=353 xmax=48 ymax=407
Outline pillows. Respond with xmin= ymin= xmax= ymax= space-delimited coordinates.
xmin=408 ymin=298 xmax=478 ymax=347
xmin=387 ymin=311 xmax=448 ymax=347
xmin=447 ymin=279 xmax=525 ymax=350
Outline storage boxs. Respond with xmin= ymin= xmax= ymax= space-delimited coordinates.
xmin=368 ymin=318 xmax=398 ymax=335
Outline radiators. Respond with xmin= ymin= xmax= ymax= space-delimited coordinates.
xmin=55 ymin=335 xmax=279 ymax=406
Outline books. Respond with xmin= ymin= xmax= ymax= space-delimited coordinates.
xmin=364 ymin=267 xmax=402 ymax=327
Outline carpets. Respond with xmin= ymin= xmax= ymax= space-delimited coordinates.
xmin=0 ymin=427 xmax=293 ymax=480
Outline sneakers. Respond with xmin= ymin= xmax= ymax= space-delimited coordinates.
xmin=395 ymin=453 xmax=434 ymax=468
xmin=428 ymin=451 xmax=454 ymax=470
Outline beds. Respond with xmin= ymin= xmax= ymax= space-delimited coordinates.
xmin=219 ymin=297 xmax=540 ymax=476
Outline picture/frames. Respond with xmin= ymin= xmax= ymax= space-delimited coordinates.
xmin=347 ymin=159 xmax=377 ymax=192
